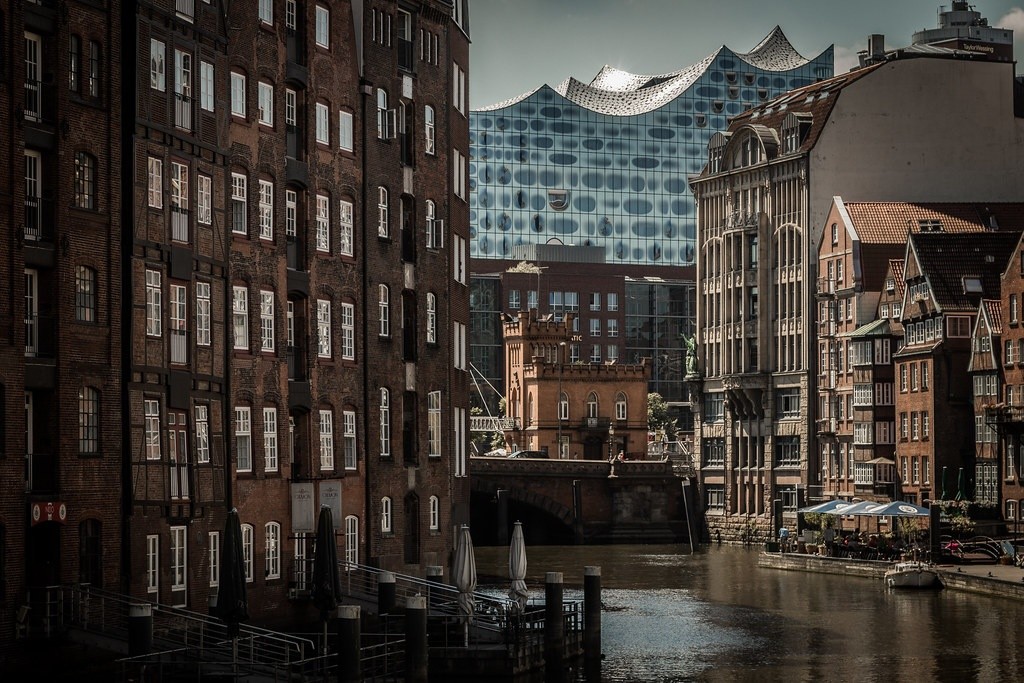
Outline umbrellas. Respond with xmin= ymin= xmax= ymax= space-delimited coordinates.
xmin=797 ymin=499 xmax=946 ymax=543
xmin=508 ymin=519 xmax=527 ymax=638
xmin=453 ymin=523 xmax=478 ymax=647
xmin=216 ymin=509 xmax=249 ymax=668
xmin=311 ymin=507 xmax=343 ymax=683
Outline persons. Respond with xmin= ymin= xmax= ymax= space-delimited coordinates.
xmin=779 ymin=523 xmax=959 ymax=562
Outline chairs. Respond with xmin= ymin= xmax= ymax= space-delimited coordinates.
xmin=835 ymin=543 xmax=900 ymax=561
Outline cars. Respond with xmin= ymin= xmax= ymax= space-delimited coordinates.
xmin=506 ymin=451 xmax=550 ymax=459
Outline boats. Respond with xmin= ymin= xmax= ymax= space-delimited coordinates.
xmin=883 ymin=530 xmax=942 ymax=588
